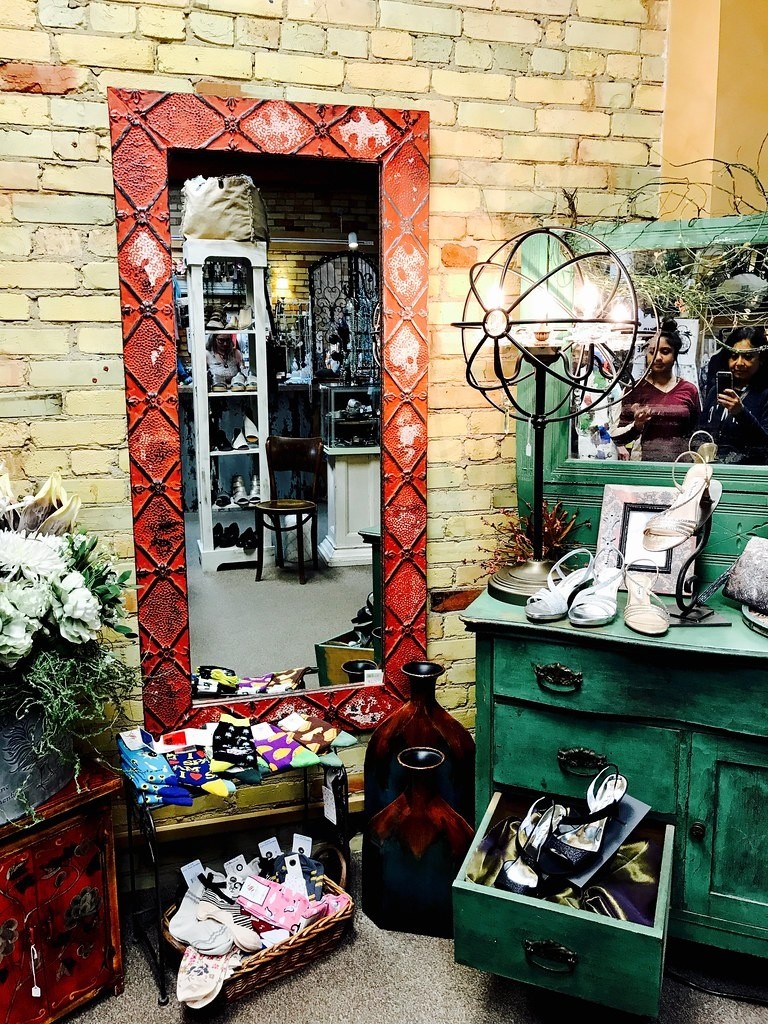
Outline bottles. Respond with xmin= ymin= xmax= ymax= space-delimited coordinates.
xmin=372 ymin=627 xmax=384 ymax=670
xmin=341 ymin=659 xmax=378 ymax=684
xmin=362 ymin=747 xmax=475 ymax=940
xmin=364 ymin=662 xmax=476 ymax=832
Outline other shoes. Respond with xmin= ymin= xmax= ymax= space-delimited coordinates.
xmin=171 ymin=255 xmax=260 ymax=553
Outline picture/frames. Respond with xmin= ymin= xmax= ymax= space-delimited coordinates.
xmin=594 ymin=484 xmax=698 ymax=596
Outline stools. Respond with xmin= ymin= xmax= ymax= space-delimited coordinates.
xmin=254 ymin=435 xmax=323 ymax=585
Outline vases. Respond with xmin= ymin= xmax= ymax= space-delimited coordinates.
xmin=363 ymin=662 xmax=474 ymax=823
xmin=0 ymin=646 xmax=80 ymax=823
xmin=361 ymin=744 xmax=473 ymax=938
xmin=341 ymin=658 xmax=378 ymax=684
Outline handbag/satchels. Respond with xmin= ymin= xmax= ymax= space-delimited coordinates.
xmin=182 ymin=173 xmax=270 ymax=243
xmin=695 ymin=532 xmax=768 ymax=612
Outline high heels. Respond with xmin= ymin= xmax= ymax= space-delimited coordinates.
xmin=524 ymin=548 xmax=596 ymax=623
xmin=623 ymin=558 xmax=670 ymax=636
xmin=688 ymin=431 xmax=718 ymax=462
xmin=642 ymin=451 xmax=714 ymax=552
xmin=567 ymin=545 xmax=625 ymax=627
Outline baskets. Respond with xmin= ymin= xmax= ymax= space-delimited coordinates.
xmin=160 ymin=841 xmax=356 ymax=1007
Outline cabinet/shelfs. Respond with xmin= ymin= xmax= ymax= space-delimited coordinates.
xmin=452 ymin=568 xmax=768 ymax=1018
xmin=181 ymin=238 xmax=271 ymax=571
xmin=0 ymin=751 xmax=123 ymax=1024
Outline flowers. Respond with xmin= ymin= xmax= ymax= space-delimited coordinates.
xmin=0 ymin=463 xmax=149 ymax=798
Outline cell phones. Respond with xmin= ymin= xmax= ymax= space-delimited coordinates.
xmin=716 ymin=372 xmax=733 ymax=403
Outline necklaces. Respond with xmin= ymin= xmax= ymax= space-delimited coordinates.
xmin=215 ymin=348 xmax=228 ymax=369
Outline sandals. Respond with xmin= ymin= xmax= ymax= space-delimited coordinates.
xmin=351 ymin=592 xmax=377 ymax=626
xmin=469 ymin=763 xmax=672 ymax=925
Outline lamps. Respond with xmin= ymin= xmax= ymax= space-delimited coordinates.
xmin=452 ymin=223 xmax=662 ymax=605
xmin=347 ymin=232 xmax=359 ymax=248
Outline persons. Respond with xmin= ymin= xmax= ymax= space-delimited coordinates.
xmin=701 ymin=326 xmax=768 ymax=466
xmin=206 ymin=333 xmax=245 ymax=387
xmin=608 ymin=317 xmax=703 ymax=464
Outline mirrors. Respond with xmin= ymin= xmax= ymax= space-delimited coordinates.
xmin=537 ymin=214 xmax=768 ymax=495
xmin=107 ymin=86 xmax=429 ymax=743
